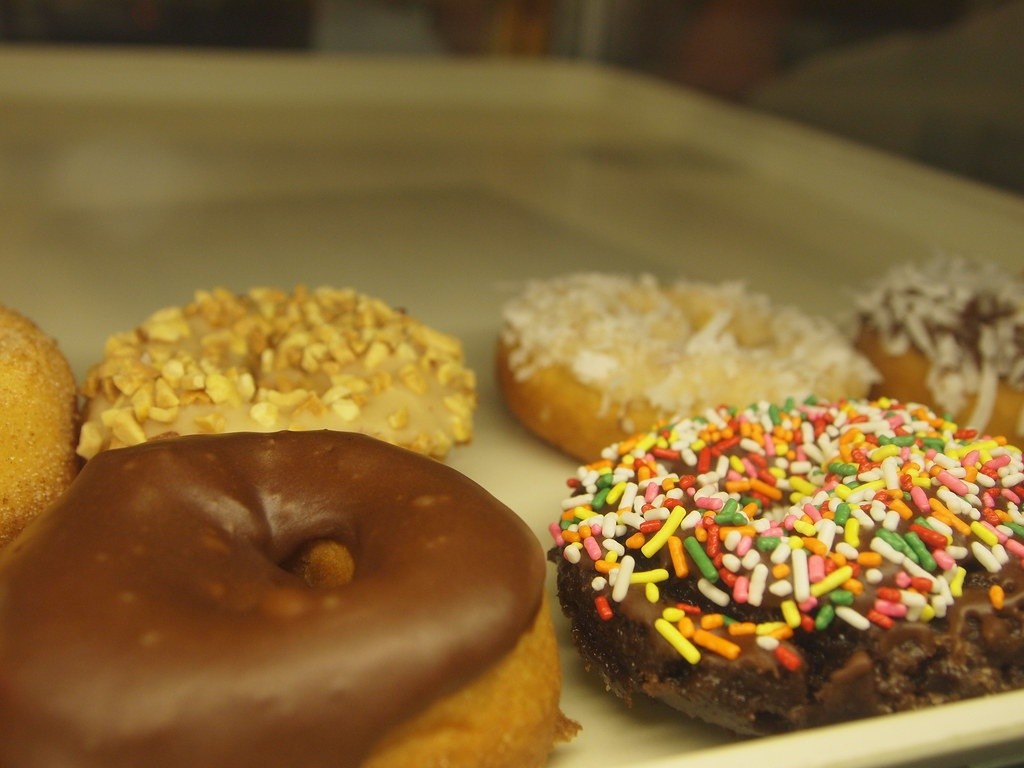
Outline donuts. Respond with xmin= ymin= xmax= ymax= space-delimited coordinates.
xmin=0 ymin=263 xmax=1024 ymax=768
